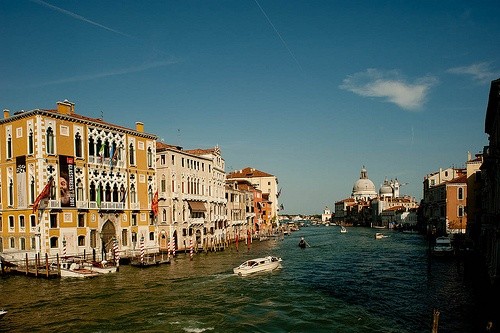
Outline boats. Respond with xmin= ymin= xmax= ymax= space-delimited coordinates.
xmin=233 ymin=256 xmax=282 ymax=276
xmin=374 ymin=233 xmax=392 ymax=239
xmin=298 ymin=236 xmax=311 ymax=248
xmin=340 ymin=225 xmax=347 ymax=233
xmin=49 ymin=258 xmax=117 ymax=279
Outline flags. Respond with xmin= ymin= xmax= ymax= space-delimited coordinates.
xmin=151 ymin=191 xmax=158 ymax=217
xmin=34 ymin=181 xmax=51 ymax=214
xmin=97 ymin=183 xmax=101 ymax=207
